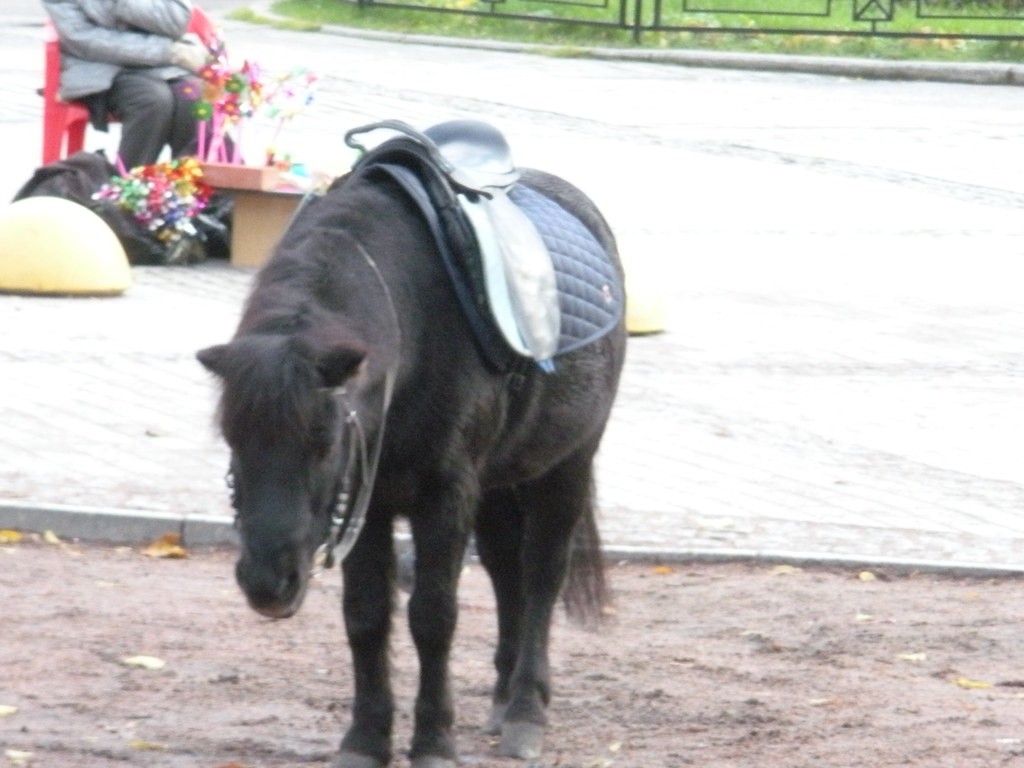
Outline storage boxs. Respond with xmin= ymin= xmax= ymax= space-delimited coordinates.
xmin=231 ymin=190 xmax=301 ymax=268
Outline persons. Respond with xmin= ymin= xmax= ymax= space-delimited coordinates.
xmin=44 ymin=0 xmax=214 ymax=173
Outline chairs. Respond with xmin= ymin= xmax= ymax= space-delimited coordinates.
xmin=42 ymin=20 xmax=122 ymax=165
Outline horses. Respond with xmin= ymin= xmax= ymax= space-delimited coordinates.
xmin=193 ymin=120 xmax=629 ymax=767
